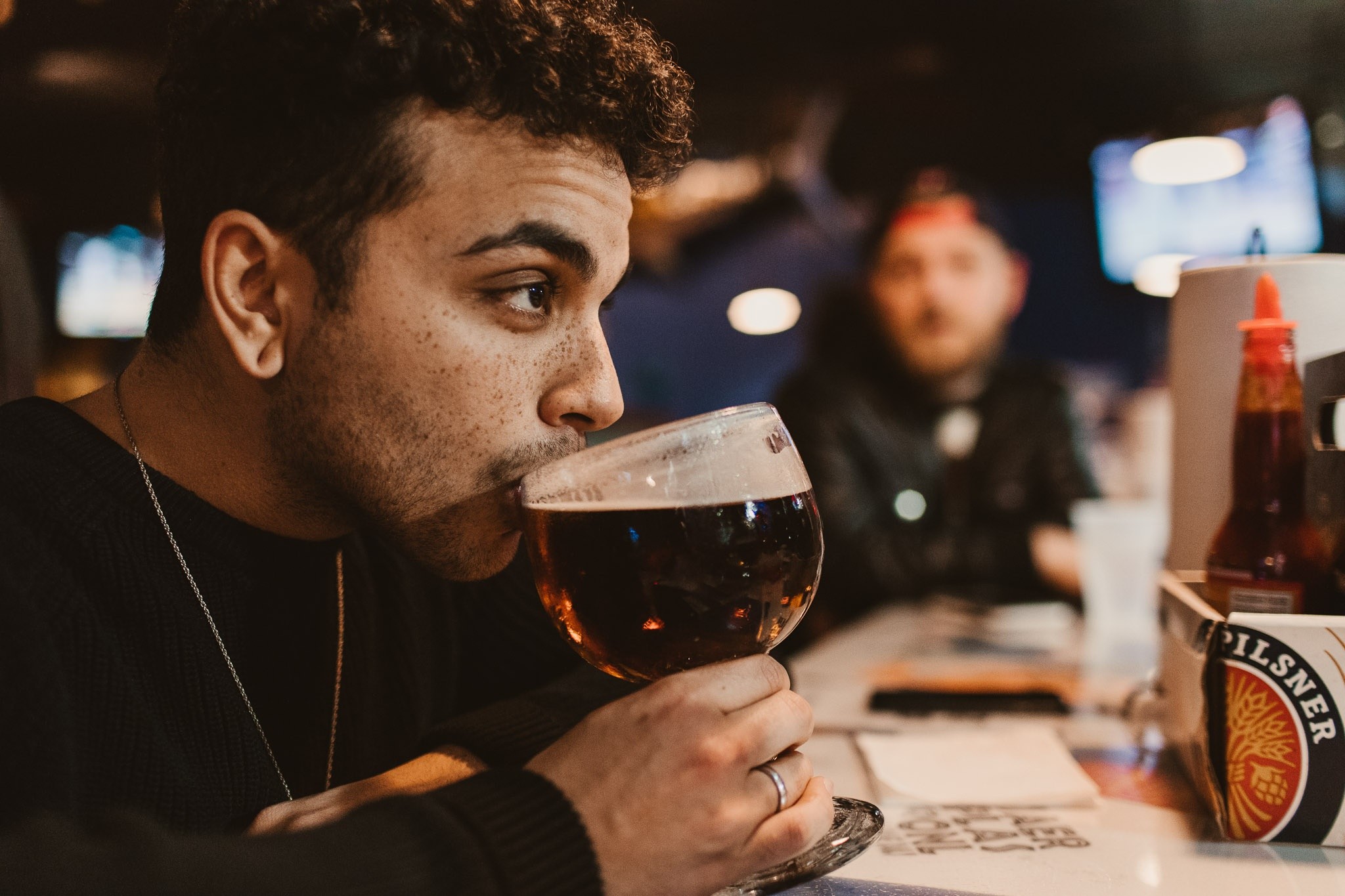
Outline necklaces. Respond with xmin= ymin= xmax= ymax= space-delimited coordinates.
xmin=115 ymin=371 xmax=346 ymax=802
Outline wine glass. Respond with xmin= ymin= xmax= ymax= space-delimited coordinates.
xmin=518 ymin=401 xmax=883 ymax=895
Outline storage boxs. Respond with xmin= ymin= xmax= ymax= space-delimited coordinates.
xmin=1159 ymin=567 xmax=1345 ymax=850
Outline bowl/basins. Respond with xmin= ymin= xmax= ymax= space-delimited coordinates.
xmin=1061 ymin=717 xmax=1183 ymax=810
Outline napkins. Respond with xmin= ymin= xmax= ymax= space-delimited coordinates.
xmin=852 ymin=728 xmax=1098 ymax=809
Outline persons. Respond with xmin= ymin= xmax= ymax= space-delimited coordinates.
xmin=0 ymin=0 xmax=838 ymax=896
xmin=774 ymin=163 xmax=1112 ymax=657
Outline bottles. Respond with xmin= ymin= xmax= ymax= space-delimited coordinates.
xmin=1204 ymin=272 xmax=1335 ymax=612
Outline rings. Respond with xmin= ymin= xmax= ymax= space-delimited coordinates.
xmin=756 ymin=764 xmax=788 ymax=812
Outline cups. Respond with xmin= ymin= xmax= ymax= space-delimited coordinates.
xmin=1071 ymin=503 xmax=1169 ymax=699
